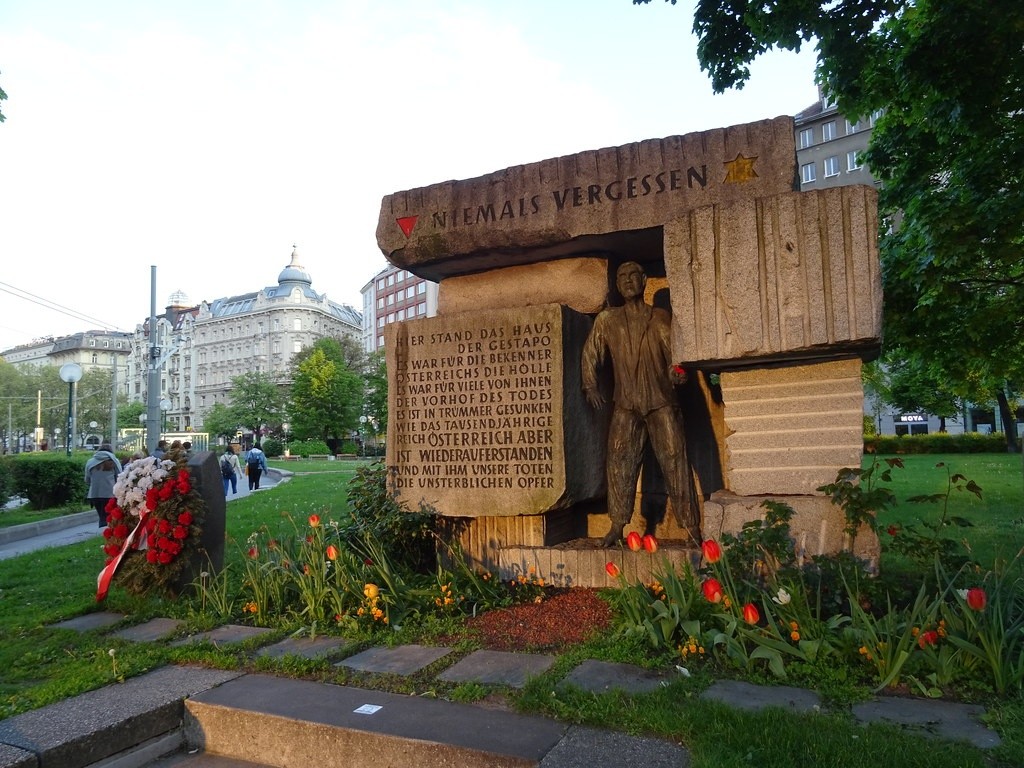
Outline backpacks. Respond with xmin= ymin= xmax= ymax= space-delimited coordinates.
xmin=222 ymin=454 xmax=235 ymax=479
xmin=248 ymin=450 xmax=262 ymax=473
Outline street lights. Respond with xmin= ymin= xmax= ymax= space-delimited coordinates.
xmin=53 ymin=427 xmax=62 ymax=448
xmin=160 ymin=399 xmax=171 ymax=442
xmin=90 ymin=421 xmax=98 ymax=450
xmin=281 ymin=422 xmax=290 ymax=452
xmin=372 ymin=423 xmax=379 ymax=460
xmin=139 ymin=412 xmax=147 ymax=453
xmin=359 ymin=416 xmax=367 ymax=458
xmin=59 ymin=363 xmax=83 ymax=457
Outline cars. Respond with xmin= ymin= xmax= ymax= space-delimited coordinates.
xmin=117 ymin=434 xmax=141 ymax=450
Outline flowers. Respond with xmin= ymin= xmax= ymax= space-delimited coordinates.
xmin=103 ymin=456 xmax=206 ymax=596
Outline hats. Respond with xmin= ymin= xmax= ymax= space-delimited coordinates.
xmin=159 ymin=441 xmax=167 ymax=447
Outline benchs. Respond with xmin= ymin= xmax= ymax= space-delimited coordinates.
xmin=279 ymin=455 xmax=301 ymax=461
xmin=337 ymin=453 xmax=357 ymax=460
xmin=309 ymin=454 xmax=329 ymax=460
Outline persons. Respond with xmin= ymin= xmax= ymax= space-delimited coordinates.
xmin=219 ymin=446 xmax=243 ymax=496
xmin=581 ymin=261 xmax=703 ymax=548
xmin=124 ymin=450 xmax=144 ymax=469
xmin=149 ymin=440 xmax=193 ymax=468
xmin=84 ymin=444 xmax=122 ymax=528
xmin=244 ymin=442 xmax=268 ymax=491
